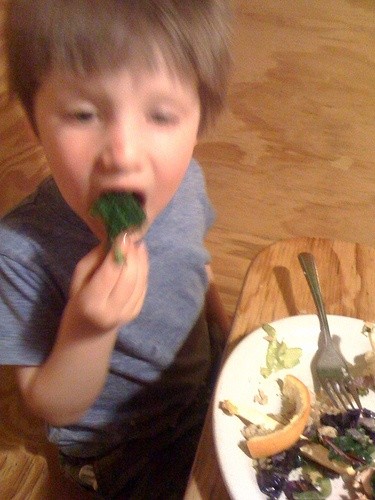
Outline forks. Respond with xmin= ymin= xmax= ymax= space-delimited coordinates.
xmin=297 ymin=252 xmax=362 ymax=414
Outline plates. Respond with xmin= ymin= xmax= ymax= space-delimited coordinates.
xmin=210 ymin=313 xmax=374 ymax=500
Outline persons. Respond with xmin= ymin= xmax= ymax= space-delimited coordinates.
xmin=0 ymin=1 xmax=232 ymax=496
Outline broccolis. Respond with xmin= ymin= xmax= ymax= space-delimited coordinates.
xmin=89 ymin=191 xmax=145 ymax=265
